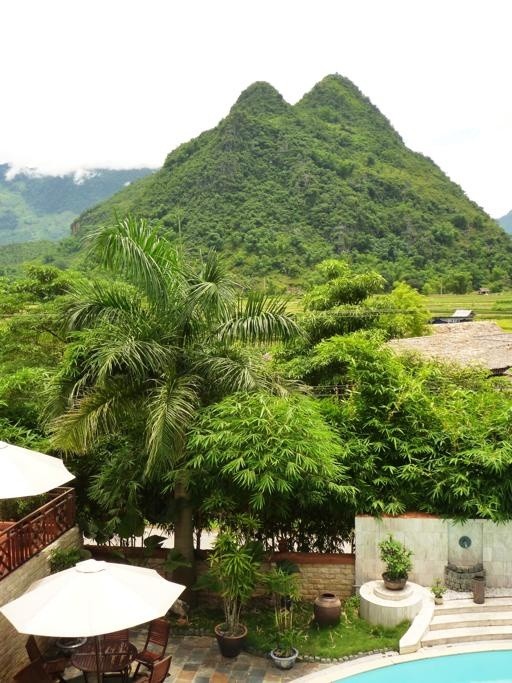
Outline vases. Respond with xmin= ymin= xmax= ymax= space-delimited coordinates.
xmin=314 ymin=593 xmax=341 ymax=628
xmin=473 ymin=577 xmax=485 ymax=605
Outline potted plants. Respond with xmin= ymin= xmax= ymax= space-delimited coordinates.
xmin=378 ymin=532 xmax=415 ymax=591
xmin=264 ymin=565 xmax=302 ymax=668
xmin=205 ymin=512 xmax=263 ymax=657
xmin=11 ymin=499 xmax=36 ymax=547
xmin=430 ymin=578 xmax=447 ymax=605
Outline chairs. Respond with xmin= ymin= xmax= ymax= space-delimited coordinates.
xmin=13 ymin=619 xmax=172 ymax=683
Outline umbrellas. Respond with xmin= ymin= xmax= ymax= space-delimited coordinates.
xmin=0 ymin=440 xmax=76 ymax=501
xmin=0 ymin=559 xmax=188 ymax=683
